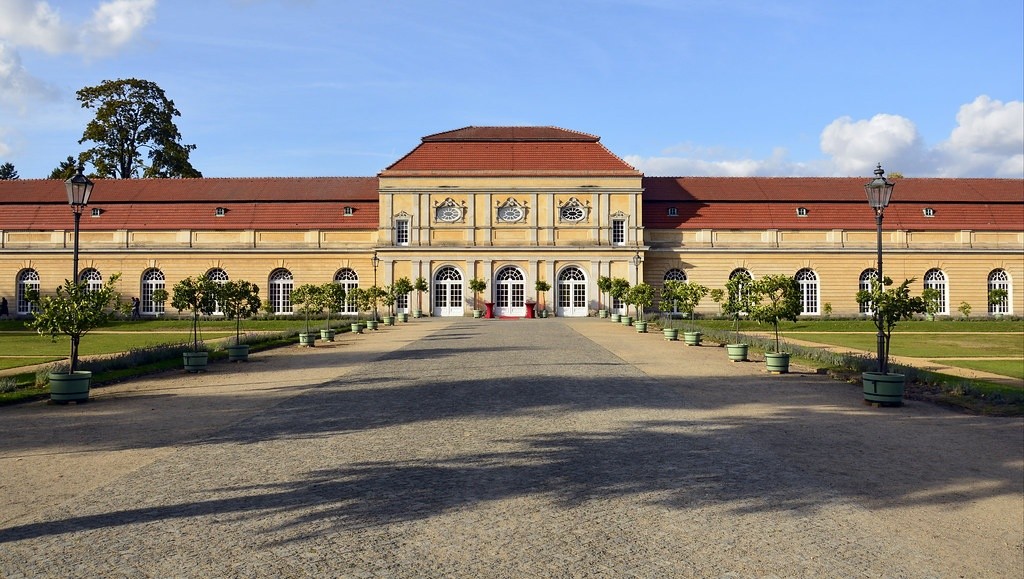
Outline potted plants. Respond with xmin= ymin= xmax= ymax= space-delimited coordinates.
xmin=365 ymin=285 xmax=389 ymax=330
xmin=608 ymin=276 xmax=630 ymax=322
xmin=534 ymin=279 xmax=553 ymax=318
xmin=676 ymin=280 xmax=712 ymax=346
xmin=22 ymin=270 xmax=133 ymax=406
xmin=344 ymin=287 xmax=372 ymax=334
xmin=628 ymin=282 xmax=656 ymax=333
xmin=468 ymin=276 xmax=491 ymax=318
xmin=216 ymin=278 xmax=262 ymax=362
xmin=378 ymin=285 xmax=398 ymax=327
xmin=596 ymin=275 xmax=612 ymax=319
xmin=391 ymin=275 xmax=431 ymax=324
xmin=658 ymin=276 xmax=692 ymax=340
xmin=169 ymin=270 xmax=220 ymax=373
xmin=854 ymin=274 xmax=1009 ymax=408
xmin=289 ymin=283 xmax=326 ymax=347
xmin=617 ymin=288 xmax=634 ymax=326
xmin=741 ymin=273 xmax=834 ymax=374
xmin=709 ymin=271 xmax=765 ymax=361
xmin=318 ymin=282 xmax=346 ymax=343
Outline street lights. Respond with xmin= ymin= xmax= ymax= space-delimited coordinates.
xmin=369 ymin=252 xmax=380 ymax=321
xmin=63 ymin=160 xmax=94 ymax=369
xmin=632 ymin=251 xmax=641 ymax=322
xmin=862 ymin=161 xmax=896 ymax=373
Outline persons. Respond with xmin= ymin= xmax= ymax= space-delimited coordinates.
xmin=131 ymin=297 xmax=140 ymax=320
xmin=0 ymin=297 xmax=9 ymax=321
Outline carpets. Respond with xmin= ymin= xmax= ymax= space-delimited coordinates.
xmin=498 ymin=315 xmax=520 ymax=320
